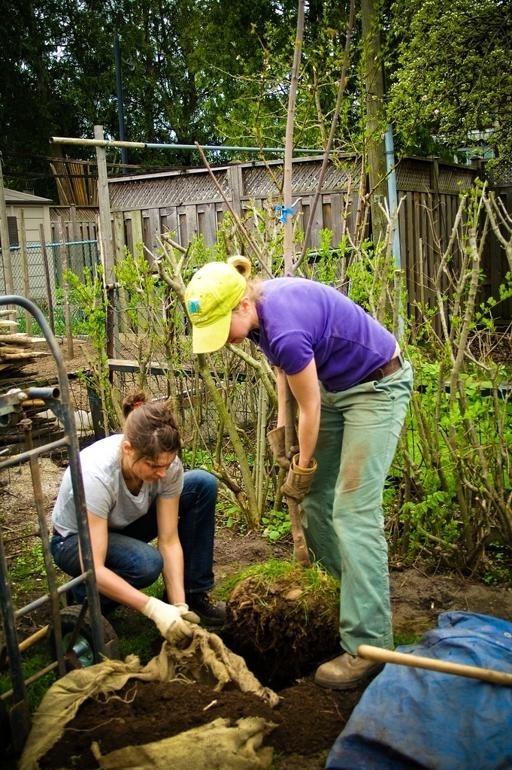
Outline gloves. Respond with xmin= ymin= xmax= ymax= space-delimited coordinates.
xmin=278 ymin=451 xmax=318 ymax=504
xmin=141 ymin=593 xmax=202 ymax=645
xmin=264 ymin=425 xmax=300 ymax=472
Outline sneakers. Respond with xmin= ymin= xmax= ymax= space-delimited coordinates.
xmin=161 ymin=585 xmax=228 ymax=627
xmin=59 ymin=588 xmax=82 ymax=609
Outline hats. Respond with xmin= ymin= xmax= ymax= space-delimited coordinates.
xmin=183 ymin=260 xmax=250 ymax=356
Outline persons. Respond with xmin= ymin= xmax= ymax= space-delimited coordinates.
xmin=48 ymin=388 xmax=227 ymax=646
xmin=182 ymin=253 xmax=414 ymax=692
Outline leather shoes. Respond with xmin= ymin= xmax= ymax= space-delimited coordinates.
xmin=314 ymin=648 xmax=388 ymax=692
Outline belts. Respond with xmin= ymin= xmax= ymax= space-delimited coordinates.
xmin=52 ymin=525 xmax=77 ymax=538
xmin=351 ymin=348 xmax=407 ymax=385
